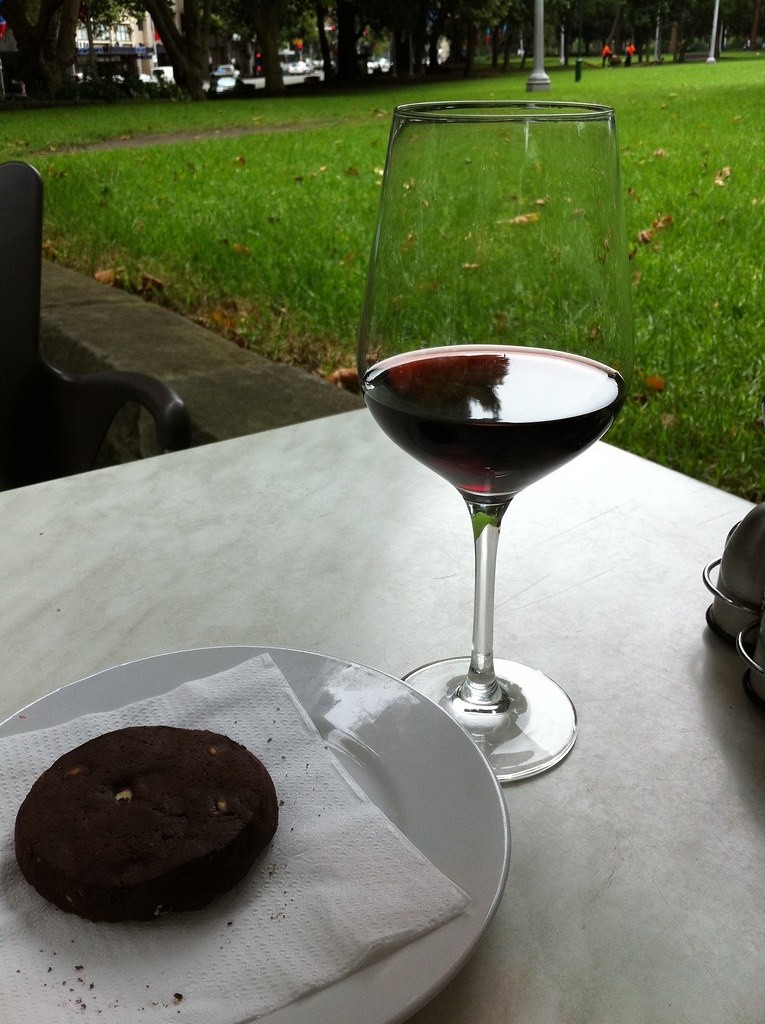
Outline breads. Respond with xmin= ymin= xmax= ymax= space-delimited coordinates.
xmin=14 ymin=725 xmax=278 ymax=923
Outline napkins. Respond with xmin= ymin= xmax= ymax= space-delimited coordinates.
xmin=0 ymin=655 xmax=474 ymax=1024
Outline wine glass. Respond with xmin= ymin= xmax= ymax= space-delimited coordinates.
xmin=358 ymin=100 xmax=633 ymax=786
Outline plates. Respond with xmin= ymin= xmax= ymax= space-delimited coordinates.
xmin=0 ymin=645 xmax=512 ymax=1024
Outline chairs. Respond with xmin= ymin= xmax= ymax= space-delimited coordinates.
xmin=1 ymin=159 xmax=219 ymax=499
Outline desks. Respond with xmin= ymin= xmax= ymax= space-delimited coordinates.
xmin=0 ymin=408 xmax=765 ymax=1024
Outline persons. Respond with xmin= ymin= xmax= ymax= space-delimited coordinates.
xmin=601 ymin=41 xmax=612 ymax=67
xmin=622 ymin=42 xmax=636 ymax=67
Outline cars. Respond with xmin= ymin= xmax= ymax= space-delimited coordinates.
xmin=288 ymin=61 xmax=314 ymax=75
xmin=210 ymin=65 xmax=241 ymax=78
